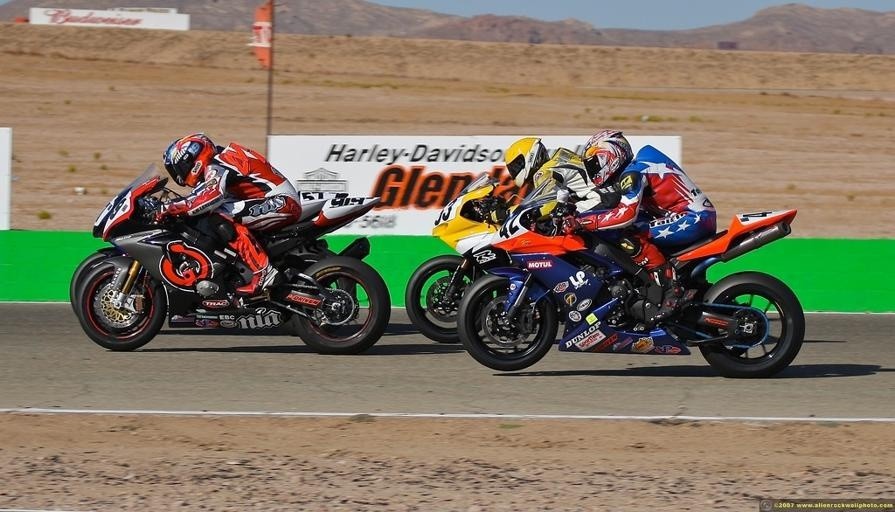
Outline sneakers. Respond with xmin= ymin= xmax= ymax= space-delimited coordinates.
xmin=236 ymin=264 xmax=278 ymax=295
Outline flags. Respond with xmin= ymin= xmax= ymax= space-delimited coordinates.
xmin=247 ymin=2 xmax=272 ymax=69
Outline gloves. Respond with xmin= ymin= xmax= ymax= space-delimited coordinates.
xmin=151 ymin=205 xmax=188 ymax=224
xmin=561 ymin=214 xmax=598 ymax=234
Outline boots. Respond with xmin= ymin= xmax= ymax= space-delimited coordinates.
xmin=647 ymin=262 xmax=698 ymax=320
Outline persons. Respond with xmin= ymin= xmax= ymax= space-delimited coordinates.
xmin=507 ymin=136 xmax=607 ymax=218
xmin=154 ymin=131 xmax=301 ymax=296
xmin=642 ymin=323 xmax=643 ymax=330
xmin=559 ymin=128 xmax=724 ymax=327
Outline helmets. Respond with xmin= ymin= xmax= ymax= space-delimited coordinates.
xmin=581 ymin=130 xmax=634 ymax=189
xmin=504 ymin=138 xmax=550 ymax=188
xmin=163 ymin=133 xmax=217 ymax=188
